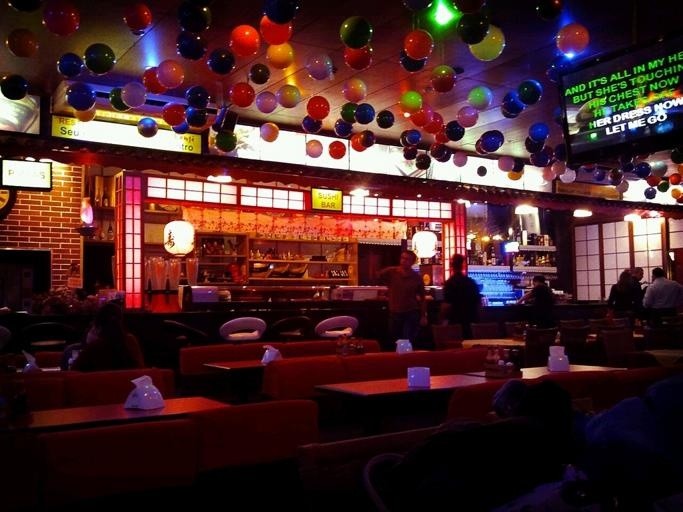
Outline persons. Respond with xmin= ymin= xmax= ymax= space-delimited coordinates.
xmin=439 ymin=253 xmax=481 ymax=340
xmin=517 ymin=276 xmax=557 ymax=329
xmin=607 ymin=267 xmax=683 ymax=331
xmin=369 ymin=250 xmax=429 ymax=352
xmin=73 ymin=303 xmax=145 ymax=372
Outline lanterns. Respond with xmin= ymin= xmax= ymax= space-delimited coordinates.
xmin=411 ymin=230 xmax=438 ymax=259
xmin=164 ymin=221 xmax=194 ymax=257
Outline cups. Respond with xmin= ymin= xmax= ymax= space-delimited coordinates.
xmin=110 ymin=254 xmax=200 ymax=290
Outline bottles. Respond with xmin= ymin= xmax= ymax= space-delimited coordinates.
xmin=478 ymin=251 xmax=483 ymax=265
xmin=433 ymin=247 xmax=441 ymax=264
xmin=248 ymin=250 xmax=253 ymax=260
xmin=483 ymin=344 xmax=524 ymax=379
xmin=406 ymin=222 xmax=429 ymax=240
xmin=201 ymin=241 xmax=224 ymax=256
xmin=530 ymin=233 xmax=537 ymax=245
xmin=489 ymin=247 xmax=496 ymax=265
xmin=281 ymin=250 xmax=290 ymax=260
xmin=335 ymin=332 xmax=370 ymax=354
xmin=102 ymin=185 xmax=110 ymax=207
xmin=255 ymin=249 xmax=260 ymax=260
xmin=0 ymin=86 xmax=39 ymax=134
xmin=514 ymin=251 xmax=553 ymax=267
xmin=95 ymin=188 xmax=102 ymax=206
xmin=107 ymin=221 xmax=114 ymax=240
xmin=480 ymin=295 xmax=488 ymax=306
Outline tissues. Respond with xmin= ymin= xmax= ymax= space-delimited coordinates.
xmin=124 ymin=374 xmax=165 ymax=410
xmin=22 ymin=350 xmax=39 ymax=372
xmin=394 ymin=339 xmax=412 ymax=353
xmin=547 ymin=345 xmax=570 ymax=372
xmin=260 ymin=345 xmax=283 ymax=364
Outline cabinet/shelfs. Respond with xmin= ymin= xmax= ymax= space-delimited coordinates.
xmin=249 ymin=237 xmax=358 ymax=285
xmin=512 ymin=244 xmax=558 ymax=297
xmin=84 ymin=175 xmax=117 ymax=244
xmin=194 ymin=232 xmax=250 ymax=287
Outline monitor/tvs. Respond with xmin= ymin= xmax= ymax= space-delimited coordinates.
xmin=557 ymin=31 xmax=683 ymax=166
xmin=0 ymin=159 xmax=52 ymax=192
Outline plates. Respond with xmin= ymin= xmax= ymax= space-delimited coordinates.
xmin=311 ymin=255 xmax=325 ymax=259
